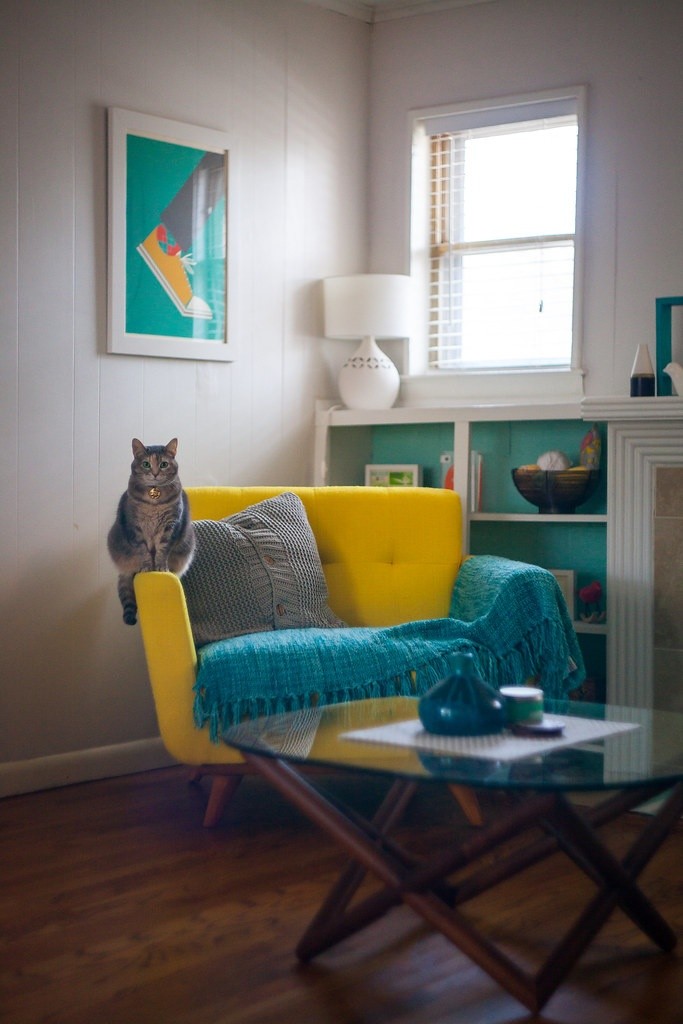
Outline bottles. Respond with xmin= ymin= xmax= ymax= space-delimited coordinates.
xmin=417 ymin=651 xmax=507 ymax=735
xmin=629 ymin=343 xmax=655 ymax=397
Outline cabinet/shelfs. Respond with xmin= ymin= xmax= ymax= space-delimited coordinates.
xmin=315 ymin=399 xmax=607 ymax=764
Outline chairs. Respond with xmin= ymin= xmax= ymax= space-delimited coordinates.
xmin=135 ymin=486 xmax=556 ymax=827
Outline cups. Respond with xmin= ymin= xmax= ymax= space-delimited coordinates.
xmin=498 ymin=685 xmax=544 ymax=726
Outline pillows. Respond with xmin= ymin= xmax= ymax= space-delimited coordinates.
xmin=180 ymin=493 xmax=346 ymax=647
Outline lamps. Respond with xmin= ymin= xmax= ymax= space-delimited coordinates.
xmin=322 ymin=274 xmax=420 ymax=410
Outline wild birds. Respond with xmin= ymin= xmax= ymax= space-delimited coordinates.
xmin=576 ymin=581 xmax=601 ymax=611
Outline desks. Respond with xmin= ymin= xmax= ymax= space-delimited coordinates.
xmin=581 ymin=397 xmax=683 ymax=816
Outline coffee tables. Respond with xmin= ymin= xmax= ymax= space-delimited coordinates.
xmin=222 ymin=696 xmax=683 ymax=1013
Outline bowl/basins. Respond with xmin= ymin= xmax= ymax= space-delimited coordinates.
xmin=511 ymin=468 xmax=600 ymax=514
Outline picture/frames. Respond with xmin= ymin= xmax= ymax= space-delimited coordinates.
xmin=108 ymin=105 xmax=238 ymax=362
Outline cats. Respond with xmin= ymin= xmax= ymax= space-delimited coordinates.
xmin=107 ymin=437 xmax=195 ymax=625
